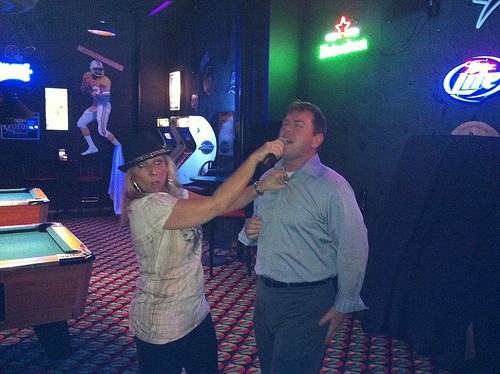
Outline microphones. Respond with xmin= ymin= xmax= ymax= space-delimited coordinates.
xmin=264 ymin=136 xmax=287 ymax=166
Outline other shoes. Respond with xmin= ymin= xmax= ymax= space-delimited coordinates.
xmin=80 ymin=147 xmax=99 ymax=155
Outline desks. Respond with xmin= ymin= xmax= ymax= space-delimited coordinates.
xmin=189 ymin=177 xmax=226 ymax=186
xmin=0 ymin=222 xmax=97 ymax=360
xmin=0 ymin=186 xmax=50 ymax=226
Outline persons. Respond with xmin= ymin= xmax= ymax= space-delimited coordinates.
xmin=77 ymin=60 xmax=120 ymax=155
xmin=118 ymin=128 xmax=289 ymax=374
xmin=239 ymin=102 xmax=369 ymax=374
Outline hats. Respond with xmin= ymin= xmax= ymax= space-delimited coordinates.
xmin=117 ymin=128 xmax=172 ymax=172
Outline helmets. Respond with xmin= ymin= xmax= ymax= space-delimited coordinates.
xmin=89 ymin=60 xmax=104 ymax=77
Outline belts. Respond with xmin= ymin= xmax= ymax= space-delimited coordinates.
xmin=259 ymin=275 xmax=332 ymax=289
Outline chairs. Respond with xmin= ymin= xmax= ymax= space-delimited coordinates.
xmin=210 ymin=199 xmax=253 ymax=276
xmin=24 ymin=159 xmax=59 ymax=216
xmin=75 ymin=153 xmax=105 ymax=219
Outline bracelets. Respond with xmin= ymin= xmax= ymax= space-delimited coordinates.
xmin=254 ymin=181 xmax=264 ymax=196
